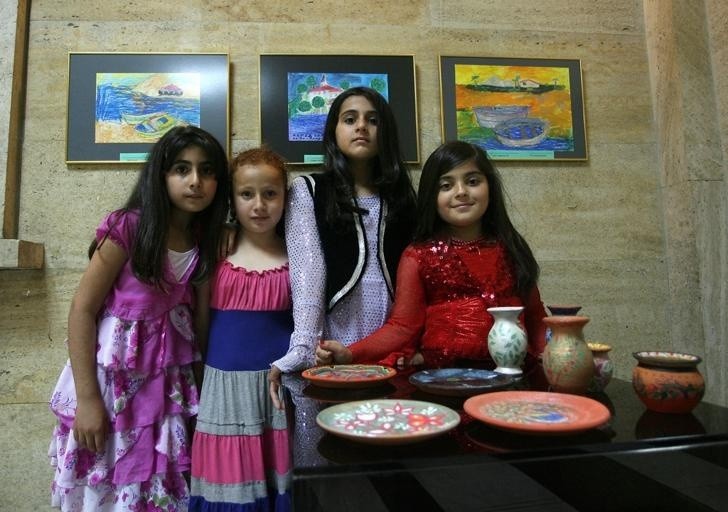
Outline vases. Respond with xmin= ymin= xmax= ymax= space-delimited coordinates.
xmin=541 ymin=315 xmax=595 ymax=397
xmin=546 ymin=303 xmax=582 ymax=315
xmin=588 ymin=343 xmax=614 ymax=393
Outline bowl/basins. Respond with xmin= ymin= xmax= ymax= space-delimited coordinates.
xmin=470 ymin=104 xmax=548 ymax=147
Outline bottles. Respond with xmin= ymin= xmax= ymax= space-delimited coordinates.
xmin=539 ymin=302 xmax=614 ymax=395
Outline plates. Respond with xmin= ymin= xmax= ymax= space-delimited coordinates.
xmin=301 ymin=364 xmax=399 ymax=389
xmin=458 ymin=419 xmax=608 ymax=452
xmin=300 ymin=383 xmax=399 ymax=404
xmin=462 ymin=390 xmax=613 ymax=435
xmin=409 ymin=367 xmax=515 ymax=395
xmin=310 ymin=431 xmax=462 ymax=464
xmin=314 ymin=400 xmax=463 ymax=446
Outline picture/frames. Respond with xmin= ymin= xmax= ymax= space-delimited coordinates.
xmin=65 ymin=50 xmax=230 ymax=165
xmin=258 ymin=51 xmax=421 ymax=167
xmin=438 ymin=53 xmax=589 ymax=163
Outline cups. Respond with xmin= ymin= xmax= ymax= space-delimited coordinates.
xmin=633 ymin=406 xmax=708 ymax=444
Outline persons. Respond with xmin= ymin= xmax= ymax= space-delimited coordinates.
xmin=266 ymin=84 xmax=418 ymax=411
xmin=187 ymin=147 xmax=293 ymax=510
xmin=314 ymin=139 xmax=549 ymax=366
xmin=50 ymin=125 xmax=231 ymax=512
